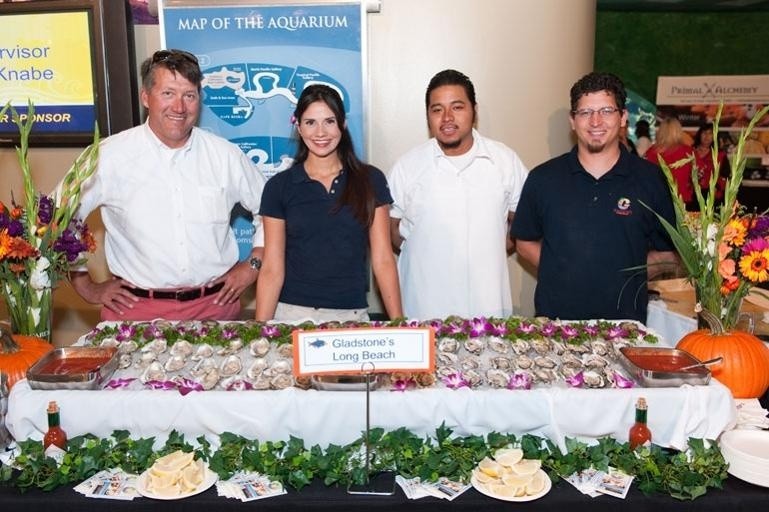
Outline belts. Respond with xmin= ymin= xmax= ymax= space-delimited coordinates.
xmin=121 ymin=283 xmax=224 ymax=300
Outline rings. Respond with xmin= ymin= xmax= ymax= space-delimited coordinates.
xmin=230 ymin=287 xmax=235 ymax=291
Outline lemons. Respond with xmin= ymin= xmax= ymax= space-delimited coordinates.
xmin=143 ymin=450 xmax=203 ymax=497
xmin=471 ymin=447 xmax=545 ymax=499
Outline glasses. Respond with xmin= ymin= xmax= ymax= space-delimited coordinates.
xmin=573 ymin=106 xmax=618 ymax=115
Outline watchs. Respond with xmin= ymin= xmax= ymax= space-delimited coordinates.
xmin=243 ymin=255 xmax=263 ymax=274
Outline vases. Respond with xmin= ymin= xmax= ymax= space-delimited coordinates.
xmin=2 ymin=280 xmax=52 ymax=346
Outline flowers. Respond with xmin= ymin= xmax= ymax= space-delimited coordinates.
xmin=0 ymin=99 xmax=99 ymax=334
xmin=639 ymin=99 xmax=769 ymax=333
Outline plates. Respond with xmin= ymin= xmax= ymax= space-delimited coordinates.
xmin=134 ymin=466 xmax=218 ymax=502
xmin=470 ymin=468 xmax=553 ymax=503
xmin=719 ymin=428 xmax=769 ymax=488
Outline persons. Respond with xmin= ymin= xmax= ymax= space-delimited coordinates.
xmin=386 ymin=66 xmax=530 ymax=326
xmin=623 ymin=117 xmax=732 ymax=213
xmin=252 ymin=82 xmax=409 ymax=327
xmin=509 ymin=71 xmax=687 ymax=330
xmin=43 ymin=47 xmax=269 ymax=323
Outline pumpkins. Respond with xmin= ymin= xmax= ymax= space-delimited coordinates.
xmin=676 ymin=303 xmax=769 ymax=398
xmin=0 ymin=329 xmax=53 ymax=390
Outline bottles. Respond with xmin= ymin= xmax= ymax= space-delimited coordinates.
xmin=630 ymin=397 xmax=651 ymax=450
xmin=43 ymin=400 xmax=66 ymax=450
